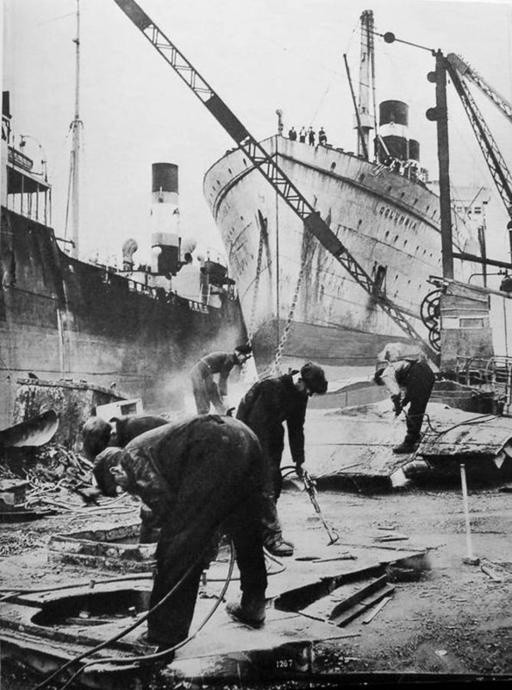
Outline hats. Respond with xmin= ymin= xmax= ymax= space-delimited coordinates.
xmin=235 ymin=345 xmax=252 ymax=353
xmin=82 ymin=416 xmax=122 ymax=496
xmin=301 ymin=361 xmax=328 ymax=394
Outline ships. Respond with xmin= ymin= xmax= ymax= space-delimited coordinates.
xmin=203 ymin=7 xmax=497 ymax=412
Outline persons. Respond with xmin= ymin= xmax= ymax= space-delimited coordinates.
xmin=83 ymin=415 xmax=170 ymax=545
xmin=91 ymin=413 xmax=267 ymax=667
xmin=289 ymin=127 xmax=297 ymax=140
xmin=236 ymin=360 xmax=328 ymax=557
xmin=318 ymin=127 xmax=328 ymax=146
xmin=307 ymin=127 xmax=315 ymax=146
xmin=374 ymin=359 xmax=434 ymax=454
xmin=298 ymin=126 xmax=307 ymax=144
xmin=190 ymin=343 xmax=253 ymax=415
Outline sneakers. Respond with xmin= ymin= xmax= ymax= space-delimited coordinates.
xmin=226 ymin=600 xmax=266 ymax=622
xmin=262 ymin=530 xmax=293 ymax=556
xmin=393 ymin=447 xmax=414 ymax=453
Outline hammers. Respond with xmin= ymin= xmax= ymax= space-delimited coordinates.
xmin=459 ymin=464 xmax=480 ymax=565
